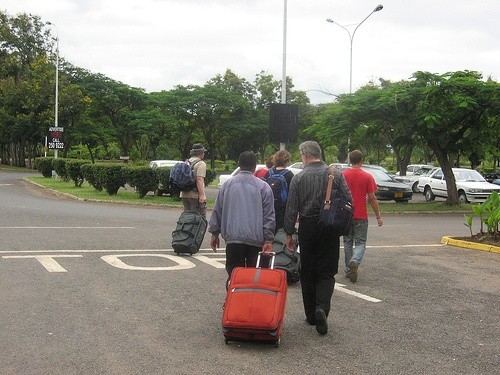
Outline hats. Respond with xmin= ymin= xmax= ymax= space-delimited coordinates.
xmin=190 ymin=143 xmax=207 ymax=154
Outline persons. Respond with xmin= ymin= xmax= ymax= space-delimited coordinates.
xmin=179 ymin=143 xmax=207 ymax=219
xmin=253 ymin=154 xmax=274 ymax=180
xmin=341 ymin=150 xmax=383 ymax=283
xmin=208 ymin=152 xmax=276 ymax=310
xmin=284 ymin=141 xmax=354 ymax=334
xmin=258 ymin=150 xmax=294 ymax=237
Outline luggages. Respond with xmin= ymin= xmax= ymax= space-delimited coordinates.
xmin=221 ymin=251 xmax=288 ymax=348
xmin=171 ymin=202 xmax=207 ymax=256
xmin=268 ymin=227 xmax=300 ymax=285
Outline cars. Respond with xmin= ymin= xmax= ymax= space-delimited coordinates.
xmin=134 ymin=160 xmax=184 ymax=195
xmin=218 ymin=162 xmax=500 ymax=205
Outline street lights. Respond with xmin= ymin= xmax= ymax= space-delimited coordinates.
xmin=45 ymin=21 xmax=59 ymax=158
xmin=325 ymin=4 xmax=384 ymax=166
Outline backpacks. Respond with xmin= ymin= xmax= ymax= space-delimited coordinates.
xmin=266 ymin=168 xmax=291 ymax=208
xmin=169 ymin=159 xmax=202 ymax=192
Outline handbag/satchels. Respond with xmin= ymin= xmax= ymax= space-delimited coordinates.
xmin=318 ymin=166 xmax=355 ymax=236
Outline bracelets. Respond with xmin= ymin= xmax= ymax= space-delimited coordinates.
xmin=376 ymin=217 xmax=381 ymax=219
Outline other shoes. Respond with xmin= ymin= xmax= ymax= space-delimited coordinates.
xmin=349 ymin=260 xmax=358 ymax=282
xmin=305 ymin=319 xmax=316 ymax=324
xmin=346 ymin=270 xmax=351 ymax=277
xmin=315 ymin=308 xmax=328 ymax=334
